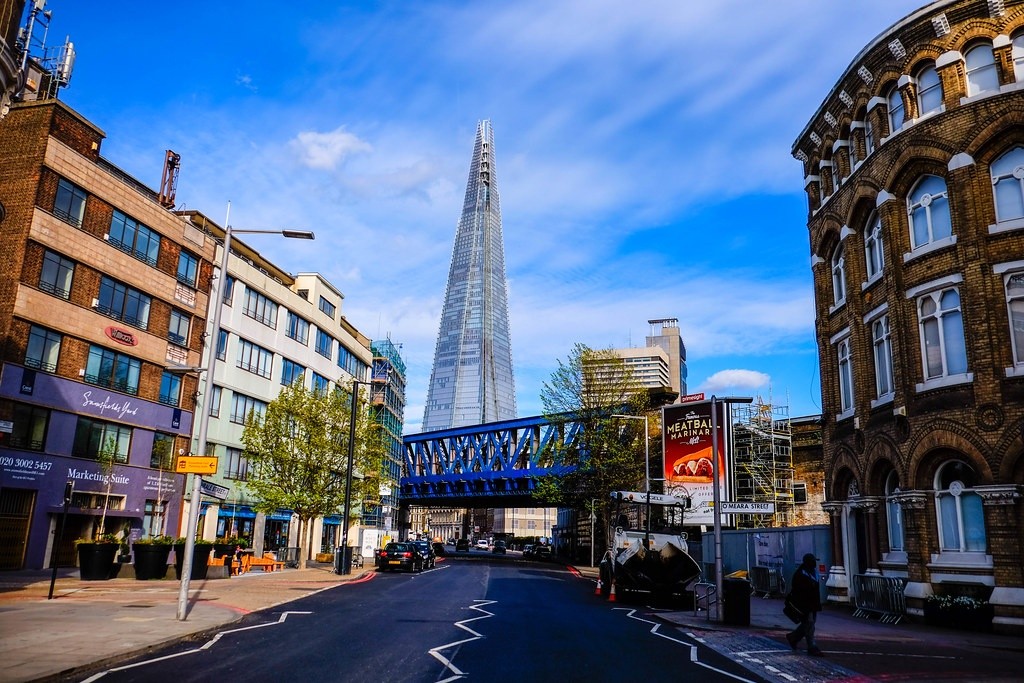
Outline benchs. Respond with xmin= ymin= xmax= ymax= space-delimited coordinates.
xmin=208 ymin=552 xmax=285 ymax=575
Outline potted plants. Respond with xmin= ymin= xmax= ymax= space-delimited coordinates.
xmin=133 ymin=533 xmax=173 ymax=581
xmin=214 ymin=535 xmax=248 ymax=576
xmin=71 ymin=534 xmax=121 ymax=581
xmin=173 ymin=537 xmax=214 ymax=581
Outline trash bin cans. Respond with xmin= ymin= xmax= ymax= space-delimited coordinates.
xmin=335 ymin=545 xmax=353 ymax=574
xmin=722 ymin=577 xmax=750 ymax=627
xmin=701 ymin=561 xmax=716 ymax=585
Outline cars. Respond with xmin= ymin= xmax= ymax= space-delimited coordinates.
xmin=378 ymin=543 xmax=424 ymax=573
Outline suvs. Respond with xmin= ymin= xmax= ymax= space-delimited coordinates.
xmin=410 ymin=541 xmax=437 ymax=570
xmin=455 ymin=538 xmax=469 ymax=552
xmin=523 ymin=544 xmax=551 ymax=561
xmin=475 ymin=540 xmax=489 ymax=551
xmin=492 ymin=540 xmax=507 ymax=555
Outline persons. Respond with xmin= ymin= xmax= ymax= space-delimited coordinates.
xmin=390 ymin=536 xmax=414 ymax=543
xmin=786 ymin=552 xmax=827 ymax=658
xmin=235 ymin=545 xmax=243 ymax=576
xmin=510 ymin=543 xmax=515 ymax=551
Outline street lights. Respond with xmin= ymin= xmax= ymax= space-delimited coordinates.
xmin=335 ymin=379 xmax=392 ymax=575
xmin=711 ymin=394 xmax=754 ymax=625
xmin=174 ymin=223 xmax=315 ymax=624
xmin=610 ymin=412 xmax=651 ymax=594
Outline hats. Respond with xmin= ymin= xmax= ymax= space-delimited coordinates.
xmin=803 ymin=553 xmax=820 ymax=561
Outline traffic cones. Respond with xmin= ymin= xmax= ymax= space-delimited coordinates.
xmin=609 ymin=584 xmax=615 ymax=602
xmin=595 ymin=576 xmax=601 ymax=595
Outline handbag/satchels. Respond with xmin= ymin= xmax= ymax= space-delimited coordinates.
xmin=783 ymin=597 xmax=809 ymax=625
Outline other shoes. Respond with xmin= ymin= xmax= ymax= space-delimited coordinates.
xmin=786 ymin=633 xmax=797 ymax=650
xmin=807 ymin=646 xmax=826 ymax=657
